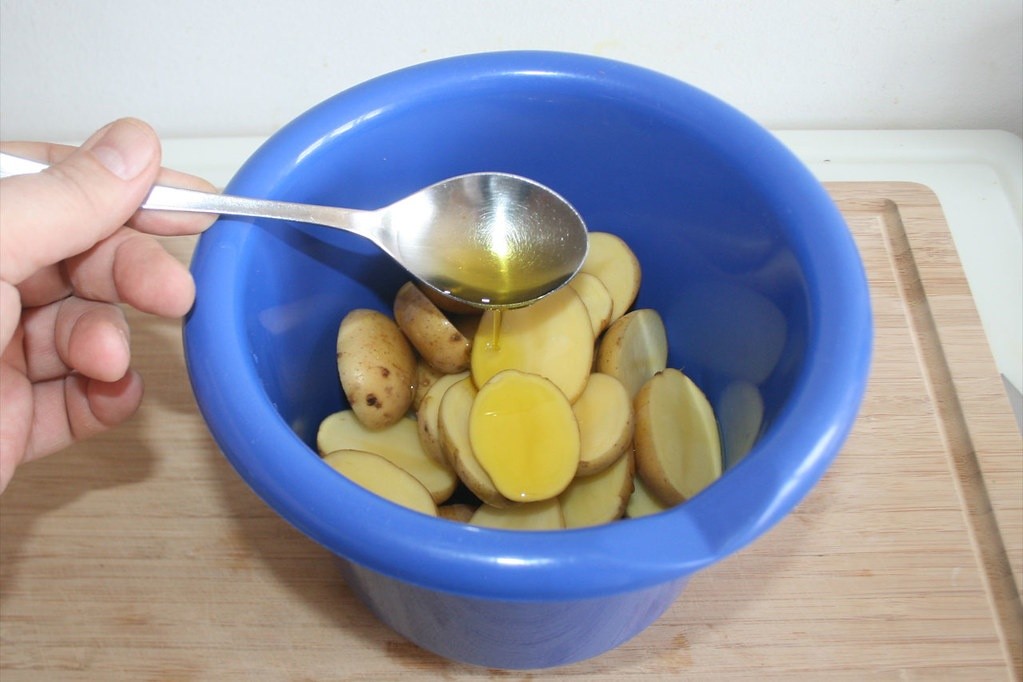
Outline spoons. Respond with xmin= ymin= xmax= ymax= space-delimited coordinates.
xmin=1 ymin=149 xmax=589 ymax=311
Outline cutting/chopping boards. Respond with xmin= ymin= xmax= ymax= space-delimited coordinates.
xmin=0 ymin=179 xmax=1023 ymax=682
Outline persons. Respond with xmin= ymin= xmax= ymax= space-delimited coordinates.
xmin=0 ymin=117 xmax=221 ymax=496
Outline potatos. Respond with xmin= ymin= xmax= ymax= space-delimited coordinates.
xmin=315 ymin=229 xmax=722 ymax=530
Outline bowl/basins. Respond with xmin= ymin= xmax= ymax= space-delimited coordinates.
xmin=179 ymin=47 xmax=874 ymax=671
